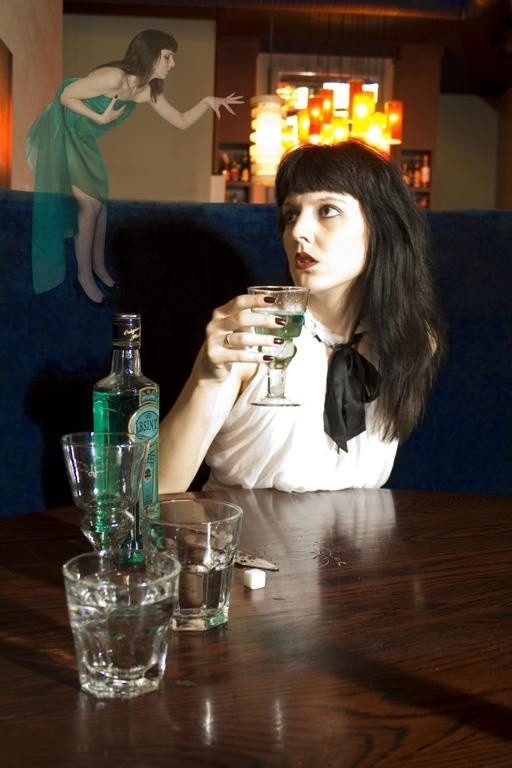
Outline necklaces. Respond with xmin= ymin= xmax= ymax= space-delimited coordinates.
xmin=299 ymin=304 xmax=360 ymax=353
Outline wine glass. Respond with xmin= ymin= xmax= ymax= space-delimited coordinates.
xmin=245 ymin=283 xmax=312 ymax=409
xmin=59 ymin=429 xmax=153 ymax=591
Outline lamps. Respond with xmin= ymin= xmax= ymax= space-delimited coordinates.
xmin=250 ymin=77 xmax=406 ymax=187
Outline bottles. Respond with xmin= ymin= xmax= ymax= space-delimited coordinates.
xmin=90 ymin=309 xmax=170 ymax=566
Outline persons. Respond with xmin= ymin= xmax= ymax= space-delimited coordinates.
xmin=158 ymin=138 xmax=445 ymax=497
xmin=24 ymin=24 xmax=248 ymax=311
xmin=158 ymin=486 xmax=427 ymax=750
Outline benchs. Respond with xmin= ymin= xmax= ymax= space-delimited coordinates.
xmin=0 ymin=189 xmax=512 ymax=514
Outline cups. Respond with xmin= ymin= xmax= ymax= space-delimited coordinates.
xmin=60 ymin=547 xmax=184 ymax=702
xmin=137 ymin=495 xmax=247 ymax=633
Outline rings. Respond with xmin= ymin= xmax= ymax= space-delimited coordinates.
xmin=225 ymin=331 xmax=238 ymax=350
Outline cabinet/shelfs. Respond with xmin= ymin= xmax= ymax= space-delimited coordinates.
xmin=391 ymin=149 xmax=438 ymax=211
xmin=211 ymin=143 xmax=252 ymax=207
